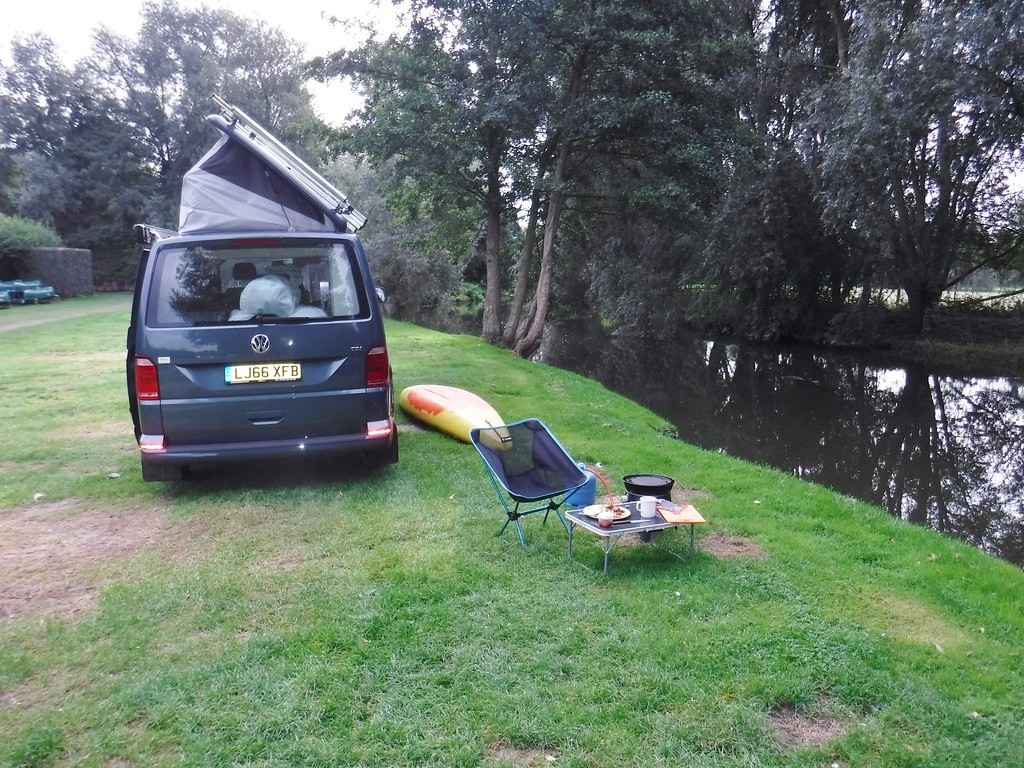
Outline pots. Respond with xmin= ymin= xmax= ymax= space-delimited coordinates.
xmin=623 ymin=474 xmax=674 ymax=495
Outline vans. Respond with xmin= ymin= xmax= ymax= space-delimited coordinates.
xmin=124 ymin=227 xmax=400 ymax=482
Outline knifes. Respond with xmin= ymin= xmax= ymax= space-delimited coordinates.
xmin=658 ymin=499 xmax=681 ymax=510
xmin=613 ymin=520 xmax=651 ymax=524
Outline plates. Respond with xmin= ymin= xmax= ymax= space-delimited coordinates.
xmin=583 ymin=505 xmax=631 ymax=520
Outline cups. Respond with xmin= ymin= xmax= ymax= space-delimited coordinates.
xmin=598 ymin=509 xmax=614 ymax=527
xmin=637 ymin=496 xmax=656 ymax=518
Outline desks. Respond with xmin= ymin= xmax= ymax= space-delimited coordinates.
xmin=565 ymin=499 xmax=694 ymax=577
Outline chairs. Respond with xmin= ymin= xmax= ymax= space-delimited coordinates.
xmin=300 ymin=263 xmax=330 ymax=305
xmin=225 ymin=262 xmax=256 ymax=305
xmin=469 ymin=418 xmax=589 ymax=550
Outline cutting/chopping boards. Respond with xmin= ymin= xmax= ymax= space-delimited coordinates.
xmin=656 ymin=505 xmax=706 ymax=523
